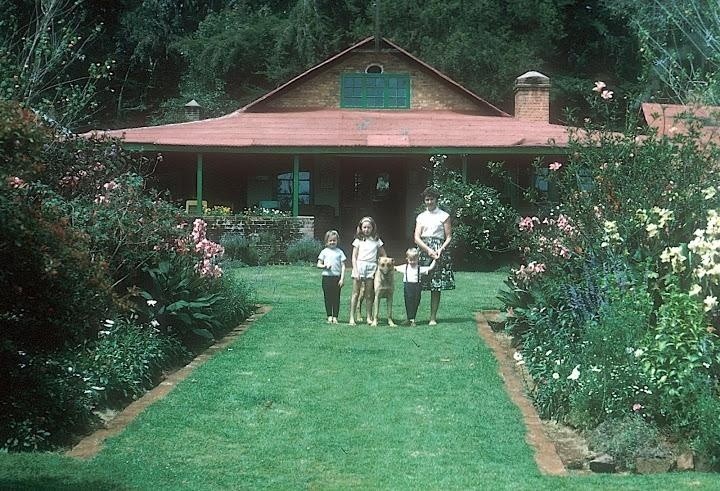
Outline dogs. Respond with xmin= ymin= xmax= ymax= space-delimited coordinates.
xmin=355 ymin=257 xmax=397 ymax=328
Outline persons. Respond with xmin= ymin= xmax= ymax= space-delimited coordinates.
xmin=349 ymin=215 xmax=388 ymax=326
xmin=316 ymin=229 xmax=347 ymax=324
xmin=400 ymin=186 xmax=456 ymax=328
xmin=392 ymin=248 xmax=436 ymax=328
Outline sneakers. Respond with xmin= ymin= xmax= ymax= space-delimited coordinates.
xmin=326 ymin=316 xmax=437 ymax=327
